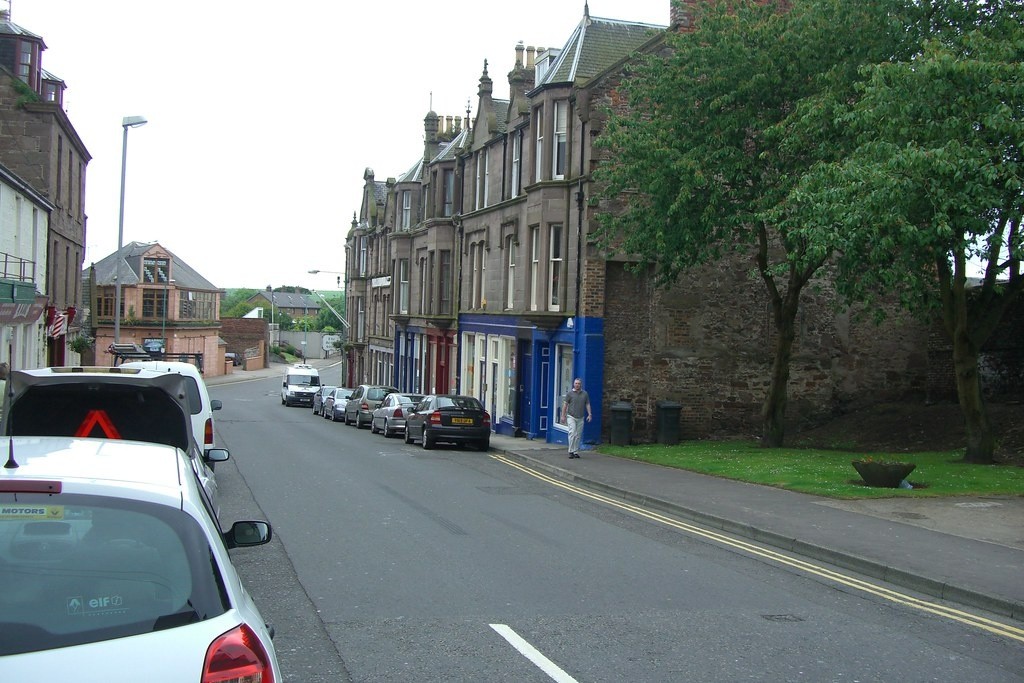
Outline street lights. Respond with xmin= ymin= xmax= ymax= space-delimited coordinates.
xmin=304 ymin=295 xmax=313 ymax=364
xmin=113 ymin=116 xmax=148 ymax=367
xmin=162 ymin=280 xmax=176 ymax=348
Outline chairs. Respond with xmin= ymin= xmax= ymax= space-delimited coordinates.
xmin=91 ymin=540 xmax=161 ymax=573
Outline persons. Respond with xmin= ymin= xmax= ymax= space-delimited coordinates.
xmin=562 ymin=378 xmax=592 ymax=458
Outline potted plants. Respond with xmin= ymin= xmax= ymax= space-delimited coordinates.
xmin=66 ymin=337 xmax=96 ymax=354
xmin=332 ymin=340 xmax=343 ymax=351
xmin=852 ymin=457 xmax=916 ymax=487
xmin=342 ymin=343 xmax=352 ymax=355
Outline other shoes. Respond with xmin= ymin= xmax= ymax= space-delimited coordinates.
xmin=569 ymin=453 xmax=574 ymax=459
xmin=574 ymin=454 xmax=580 ymax=458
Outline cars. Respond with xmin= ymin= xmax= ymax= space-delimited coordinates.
xmin=344 ymin=384 xmax=401 ymax=429
xmin=1 ymin=365 xmax=230 ymax=543
xmin=225 ymin=353 xmax=242 ymax=366
xmin=0 ymin=436 xmax=283 ymax=683
xmin=312 ymin=386 xmax=339 ymax=417
xmin=404 ymin=394 xmax=490 ymax=451
xmin=323 ymin=388 xmax=356 ymax=421
xmin=371 ymin=392 xmax=427 ymax=438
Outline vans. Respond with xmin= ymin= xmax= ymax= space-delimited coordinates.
xmin=108 ymin=343 xmax=223 ymax=472
xmin=281 ymin=364 xmax=326 ymax=408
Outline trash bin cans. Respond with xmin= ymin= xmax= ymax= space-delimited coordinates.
xmin=609 ymin=402 xmax=634 ymax=446
xmin=654 ymin=401 xmax=683 ymax=445
